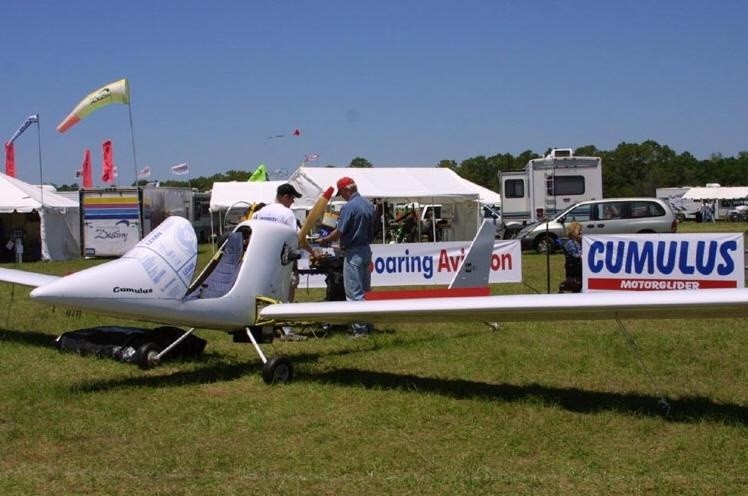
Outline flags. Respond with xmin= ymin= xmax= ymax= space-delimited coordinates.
xmin=171 ymin=162 xmax=189 ymax=175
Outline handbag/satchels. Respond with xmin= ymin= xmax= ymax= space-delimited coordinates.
xmin=6 ymin=240 xmax=15 ymax=250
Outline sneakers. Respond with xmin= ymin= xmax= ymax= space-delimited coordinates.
xmin=280 ymin=334 xmax=308 ymax=341
xmin=350 ymin=331 xmax=369 ymax=337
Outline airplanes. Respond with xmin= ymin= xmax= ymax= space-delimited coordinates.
xmin=0 ymin=208 xmax=726 ymax=386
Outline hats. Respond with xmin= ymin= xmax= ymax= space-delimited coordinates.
xmin=277 ymin=184 xmax=302 ymax=198
xmin=335 ymin=177 xmax=355 ymax=196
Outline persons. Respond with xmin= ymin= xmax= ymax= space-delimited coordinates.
xmin=312 ymin=177 xmax=377 ymax=338
xmin=251 ymin=182 xmax=323 ymax=341
xmin=562 ymin=221 xmax=583 ymax=292
xmin=421 ymin=210 xmax=439 ymax=241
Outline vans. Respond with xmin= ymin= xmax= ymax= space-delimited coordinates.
xmin=517 ymin=195 xmax=676 ymax=255
